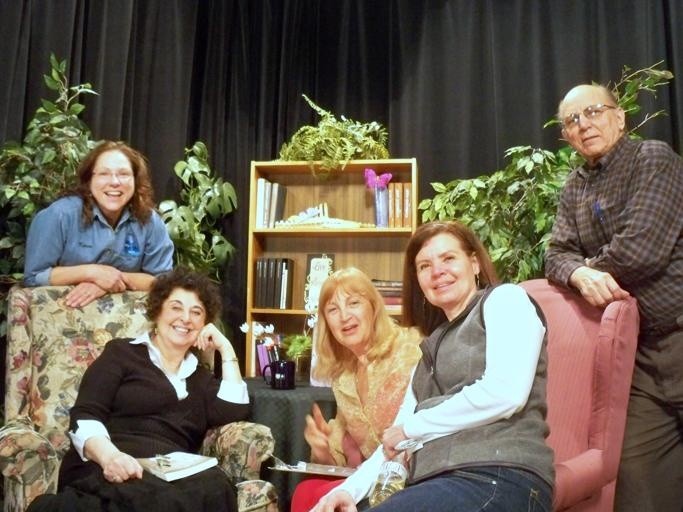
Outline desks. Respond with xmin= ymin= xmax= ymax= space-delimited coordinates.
xmin=240 ymin=373 xmax=336 ymax=511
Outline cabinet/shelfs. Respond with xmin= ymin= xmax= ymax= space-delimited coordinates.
xmin=244 ymin=157 xmax=420 ymax=380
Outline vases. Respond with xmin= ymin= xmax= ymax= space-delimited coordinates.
xmin=288 ymin=354 xmax=310 ymax=375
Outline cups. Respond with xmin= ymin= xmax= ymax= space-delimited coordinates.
xmin=261 ymin=359 xmax=297 ymax=391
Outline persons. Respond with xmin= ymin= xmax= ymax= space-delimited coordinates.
xmin=22 ymin=141 xmax=175 ymax=310
xmin=544 ymin=85 xmax=683 ymax=512
xmin=308 ymin=222 xmax=553 ymax=512
xmin=289 ymin=266 xmax=425 ymax=512
xmin=24 ymin=264 xmax=252 ymax=512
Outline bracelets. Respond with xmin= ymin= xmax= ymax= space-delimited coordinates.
xmin=220 ymin=355 xmax=241 ymax=365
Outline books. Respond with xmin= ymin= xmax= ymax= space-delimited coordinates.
xmin=255 ymin=333 xmax=284 ymax=380
xmin=137 ymin=449 xmax=219 ymax=481
xmin=368 ymin=275 xmax=404 ymax=307
xmin=255 ymin=257 xmax=294 ymax=310
xmin=387 ymin=180 xmax=412 ymax=228
xmin=256 ymin=178 xmax=287 ymax=230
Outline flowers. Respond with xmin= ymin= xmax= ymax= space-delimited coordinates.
xmin=236 ymin=310 xmax=318 ymax=362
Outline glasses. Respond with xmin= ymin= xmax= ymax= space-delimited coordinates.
xmin=563 ymin=103 xmax=617 ymax=129
xmin=90 ymin=171 xmax=134 ymax=180
xmin=155 ymin=453 xmax=172 ymax=473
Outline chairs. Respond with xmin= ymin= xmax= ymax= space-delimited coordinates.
xmin=516 ymin=278 xmax=643 ymax=511
xmin=0 ymin=282 xmax=281 ymax=511
